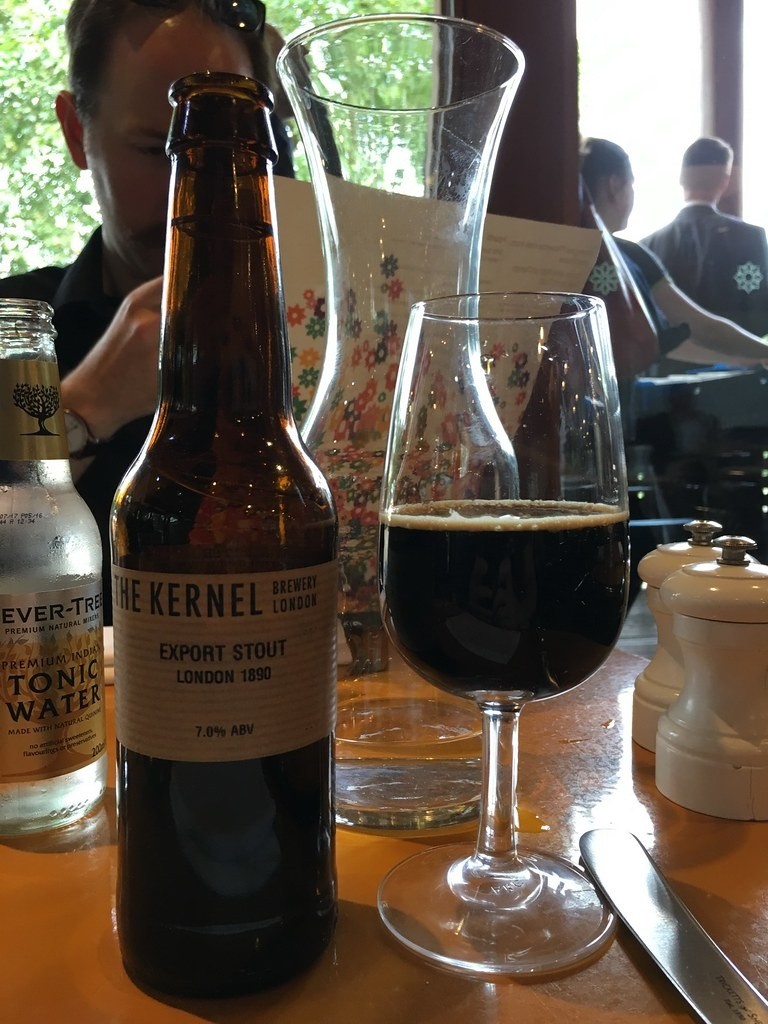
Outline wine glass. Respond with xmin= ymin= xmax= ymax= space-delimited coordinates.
xmin=381 ymin=293 xmax=628 ymax=976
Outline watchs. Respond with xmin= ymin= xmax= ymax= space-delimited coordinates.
xmin=62 ymin=409 xmax=109 ymax=459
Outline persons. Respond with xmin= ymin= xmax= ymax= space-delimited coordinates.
xmin=580 ymin=137 xmax=768 ymax=364
xmin=0 ymin=1 xmax=267 ymax=633
xmin=634 ymin=137 xmax=768 ymax=340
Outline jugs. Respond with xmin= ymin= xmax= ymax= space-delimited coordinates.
xmin=275 ymin=16 xmax=529 ymax=831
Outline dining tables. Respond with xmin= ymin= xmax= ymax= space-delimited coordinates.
xmin=1 ymin=627 xmax=768 ymax=1024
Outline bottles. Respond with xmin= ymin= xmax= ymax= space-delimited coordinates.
xmin=108 ymin=68 xmax=337 ymax=1001
xmin=0 ymin=291 xmax=102 ymax=833
